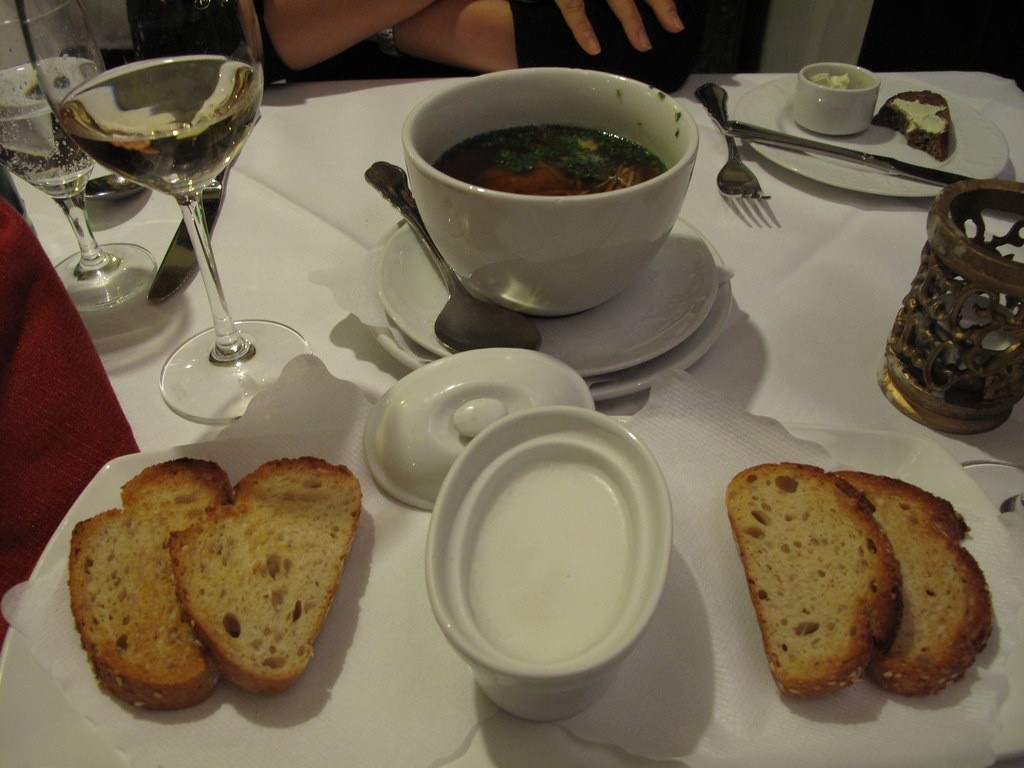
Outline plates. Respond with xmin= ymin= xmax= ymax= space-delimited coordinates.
xmin=0 ymin=414 xmax=1024 ymax=768
xmin=733 ymin=73 xmax=1009 ymax=197
xmin=367 ymin=218 xmax=733 ymax=404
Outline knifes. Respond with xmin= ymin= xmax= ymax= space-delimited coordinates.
xmin=148 ymin=164 xmax=229 ymax=302
xmin=722 ymin=121 xmax=976 ymax=187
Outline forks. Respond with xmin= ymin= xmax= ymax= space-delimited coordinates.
xmin=696 ymin=83 xmax=770 ymax=199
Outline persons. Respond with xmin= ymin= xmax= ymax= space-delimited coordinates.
xmin=235 ymin=0 xmax=703 ymax=93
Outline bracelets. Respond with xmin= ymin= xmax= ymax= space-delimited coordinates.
xmin=379 ymin=25 xmax=410 ymax=59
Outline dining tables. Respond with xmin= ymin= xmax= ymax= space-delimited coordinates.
xmin=0 ymin=73 xmax=1024 ymax=768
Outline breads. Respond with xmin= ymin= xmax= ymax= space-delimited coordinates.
xmin=827 ymin=469 xmax=993 ymax=695
xmin=70 ymin=456 xmax=236 ymax=711
xmin=168 ymin=453 xmax=363 ymax=691
xmin=871 ymin=89 xmax=951 ymax=162
xmin=724 ymin=461 xmax=901 ymax=699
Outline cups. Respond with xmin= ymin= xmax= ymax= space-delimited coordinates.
xmin=794 ymin=62 xmax=881 ymax=136
xmin=425 ymin=406 xmax=673 ymax=722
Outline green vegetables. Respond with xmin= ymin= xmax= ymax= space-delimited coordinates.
xmin=452 ymin=125 xmax=669 ymax=190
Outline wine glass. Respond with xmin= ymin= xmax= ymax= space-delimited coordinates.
xmin=16 ymin=0 xmax=312 ymax=426
xmin=1 ymin=0 xmax=157 ymax=312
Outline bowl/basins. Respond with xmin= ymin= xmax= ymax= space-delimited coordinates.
xmin=403 ymin=67 xmax=700 ymax=318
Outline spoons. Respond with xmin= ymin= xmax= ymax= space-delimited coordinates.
xmin=365 ymin=161 xmax=540 ymax=355
xmin=85 ymin=174 xmax=145 ymax=201
